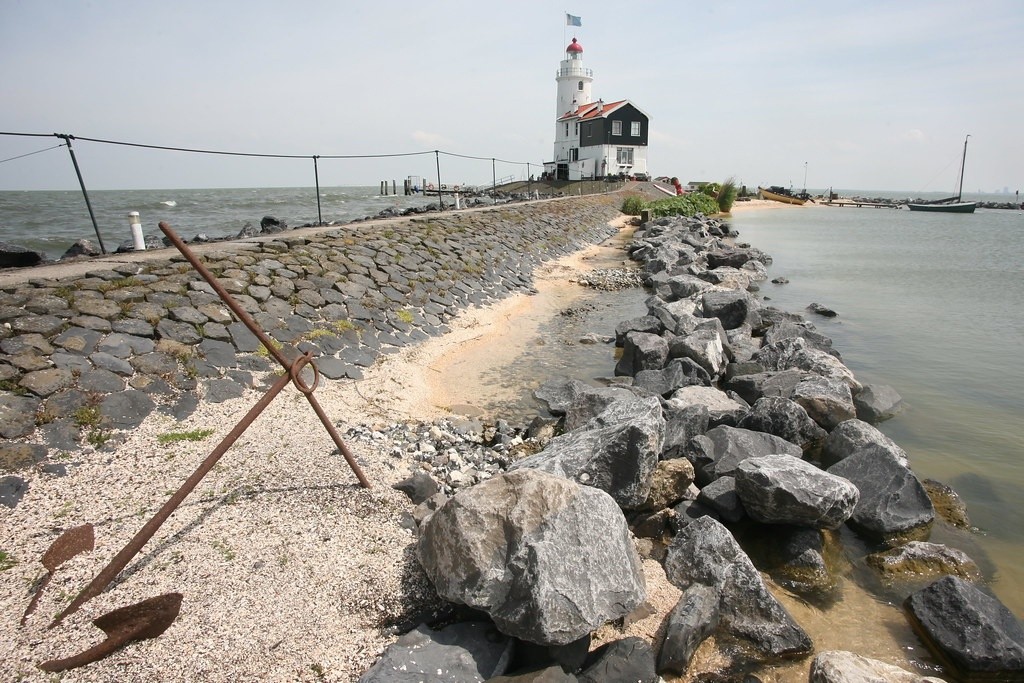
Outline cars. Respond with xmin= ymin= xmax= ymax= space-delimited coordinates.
xmin=634 ymin=172 xmax=646 ymax=181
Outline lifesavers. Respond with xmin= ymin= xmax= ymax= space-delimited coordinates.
xmin=442 ymin=184 xmax=446 ymax=190
xmin=454 ymin=186 xmax=459 ymax=191
xmin=429 ymin=184 xmax=434 ymax=190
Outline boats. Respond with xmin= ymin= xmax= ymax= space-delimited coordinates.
xmin=758 ymin=186 xmax=810 ymax=205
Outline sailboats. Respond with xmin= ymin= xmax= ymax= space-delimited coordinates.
xmin=907 ymin=134 xmax=976 ymax=213
